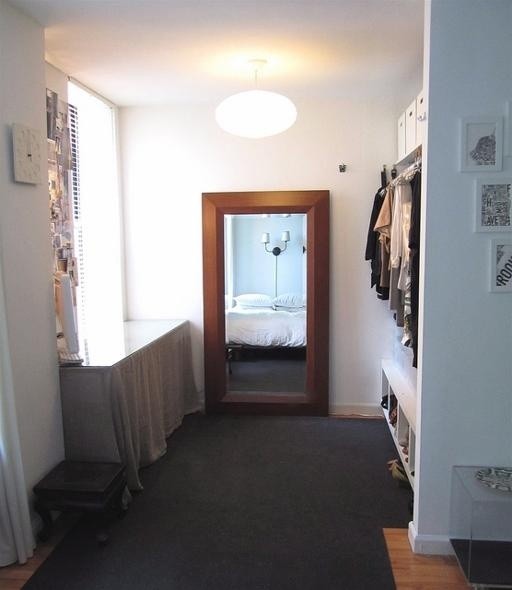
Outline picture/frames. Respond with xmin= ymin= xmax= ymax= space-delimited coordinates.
xmin=490 ymin=239 xmax=512 ymax=292
xmin=473 ymin=177 xmax=512 ymax=233
xmin=460 ymin=114 xmax=504 ymax=174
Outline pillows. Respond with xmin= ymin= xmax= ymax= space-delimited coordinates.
xmin=274 ymin=295 xmax=308 ymax=309
xmin=233 ymin=294 xmax=274 ymax=309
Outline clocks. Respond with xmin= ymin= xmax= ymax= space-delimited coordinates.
xmin=11 ymin=123 xmax=44 ymax=185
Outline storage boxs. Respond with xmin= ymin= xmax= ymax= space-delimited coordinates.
xmin=397 ymin=113 xmax=405 ymax=160
xmin=404 ymin=101 xmax=416 ymax=158
xmin=416 ymin=92 xmax=425 ymax=149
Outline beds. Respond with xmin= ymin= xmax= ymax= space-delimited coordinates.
xmin=226 ymin=296 xmax=307 ymax=374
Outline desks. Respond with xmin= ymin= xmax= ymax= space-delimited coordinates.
xmin=61 ymin=317 xmax=202 ymax=465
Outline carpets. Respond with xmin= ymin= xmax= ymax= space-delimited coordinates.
xmin=20 ymin=411 xmax=414 ymax=590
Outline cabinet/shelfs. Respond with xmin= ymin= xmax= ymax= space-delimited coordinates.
xmin=380 ymin=357 xmax=416 ymax=492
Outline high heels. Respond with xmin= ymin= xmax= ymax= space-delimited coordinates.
xmin=392 ymin=463 xmax=410 ymax=487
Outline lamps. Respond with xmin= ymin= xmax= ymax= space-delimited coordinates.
xmin=262 ymin=230 xmax=290 ymax=299
xmin=214 ymin=59 xmax=297 ymax=138
